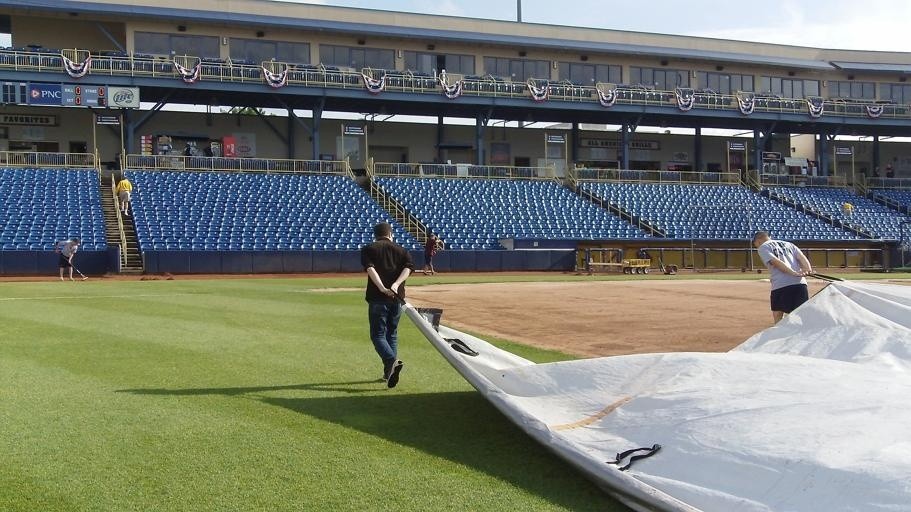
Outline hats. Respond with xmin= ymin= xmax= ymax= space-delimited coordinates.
xmin=73 ymin=238 xmax=80 ymax=246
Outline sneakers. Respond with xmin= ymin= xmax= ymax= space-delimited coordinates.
xmin=423 ymin=272 xmax=436 ymax=276
xmin=121 ymin=209 xmax=128 ymax=216
xmin=60 ymin=278 xmax=73 ymax=281
xmin=383 ymin=359 xmax=404 ymax=389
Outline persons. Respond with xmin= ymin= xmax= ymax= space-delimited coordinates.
xmin=184 ymin=143 xmax=194 ymax=168
xmin=842 ymin=200 xmax=855 ymax=220
xmin=885 ymin=163 xmax=896 ymax=186
xmin=423 ymin=232 xmax=444 ymax=277
xmin=871 ymin=165 xmax=881 ymax=185
xmin=116 ymin=177 xmax=134 ymax=215
xmin=359 ymin=221 xmax=417 ymax=389
xmin=753 ymin=229 xmax=817 ymax=326
xmin=55 ymin=237 xmax=80 ymax=283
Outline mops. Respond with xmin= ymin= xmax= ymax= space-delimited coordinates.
xmin=57 ymin=252 xmax=90 ymax=282
